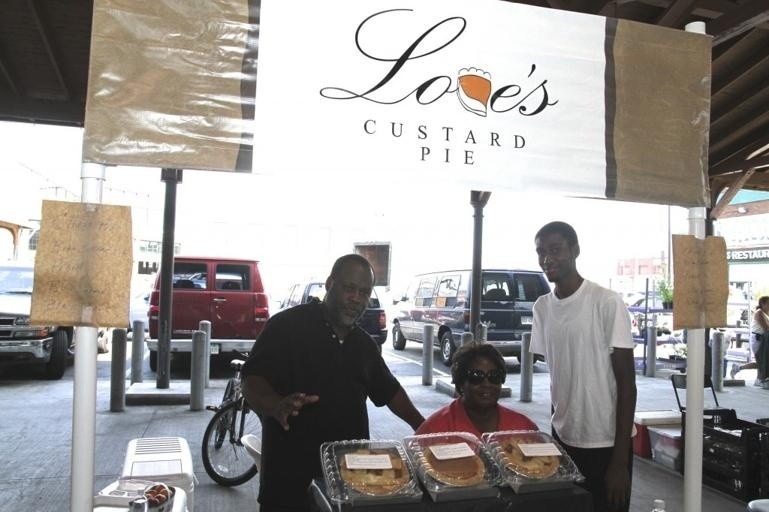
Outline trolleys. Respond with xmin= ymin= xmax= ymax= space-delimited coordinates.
xmin=671 ymin=374 xmax=769 ymax=499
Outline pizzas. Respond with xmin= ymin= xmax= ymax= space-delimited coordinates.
xmin=500 ymin=438 xmax=556 ymax=477
xmin=424 ymin=443 xmax=485 ymax=485
xmin=340 ymin=448 xmax=410 ymax=494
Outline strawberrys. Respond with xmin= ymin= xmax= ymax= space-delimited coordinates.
xmin=146 ymin=485 xmax=169 ymax=504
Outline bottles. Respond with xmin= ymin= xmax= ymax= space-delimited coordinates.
xmin=651 ymin=499 xmax=666 ymax=512
xmin=130 ymin=488 xmax=148 ymax=512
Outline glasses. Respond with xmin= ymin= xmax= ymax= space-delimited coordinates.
xmin=464 ymin=369 xmax=503 ymax=384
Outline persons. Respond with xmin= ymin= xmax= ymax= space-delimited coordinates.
xmin=239 ymin=254 xmax=428 ymax=512
xmin=730 ymin=295 xmax=768 ymax=392
xmin=413 ymin=341 xmax=547 ymax=454
xmin=527 ymin=221 xmax=636 ymax=507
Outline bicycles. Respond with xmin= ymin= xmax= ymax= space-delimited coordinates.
xmin=201 ymin=349 xmax=266 ymax=487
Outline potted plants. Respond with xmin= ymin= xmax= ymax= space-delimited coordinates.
xmin=655 ymin=281 xmax=673 ymax=309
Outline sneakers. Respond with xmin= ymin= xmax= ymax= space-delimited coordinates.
xmin=755 ymin=378 xmax=768 ymax=388
xmin=730 ymin=364 xmax=740 ymax=377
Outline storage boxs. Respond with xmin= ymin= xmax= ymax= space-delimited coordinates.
xmin=121 ymin=437 xmax=199 ymax=512
xmin=631 ymin=409 xmax=682 ymax=471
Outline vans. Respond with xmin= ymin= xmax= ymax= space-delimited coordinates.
xmin=146 ymin=256 xmax=270 ymax=371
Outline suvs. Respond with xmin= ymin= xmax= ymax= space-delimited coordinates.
xmin=392 ymin=268 xmax=552 ymax=367
xmin=0 ymin=265 xmax=76 ymax=381
xmin=280 ymin=281 xmax=388 ymax=354
